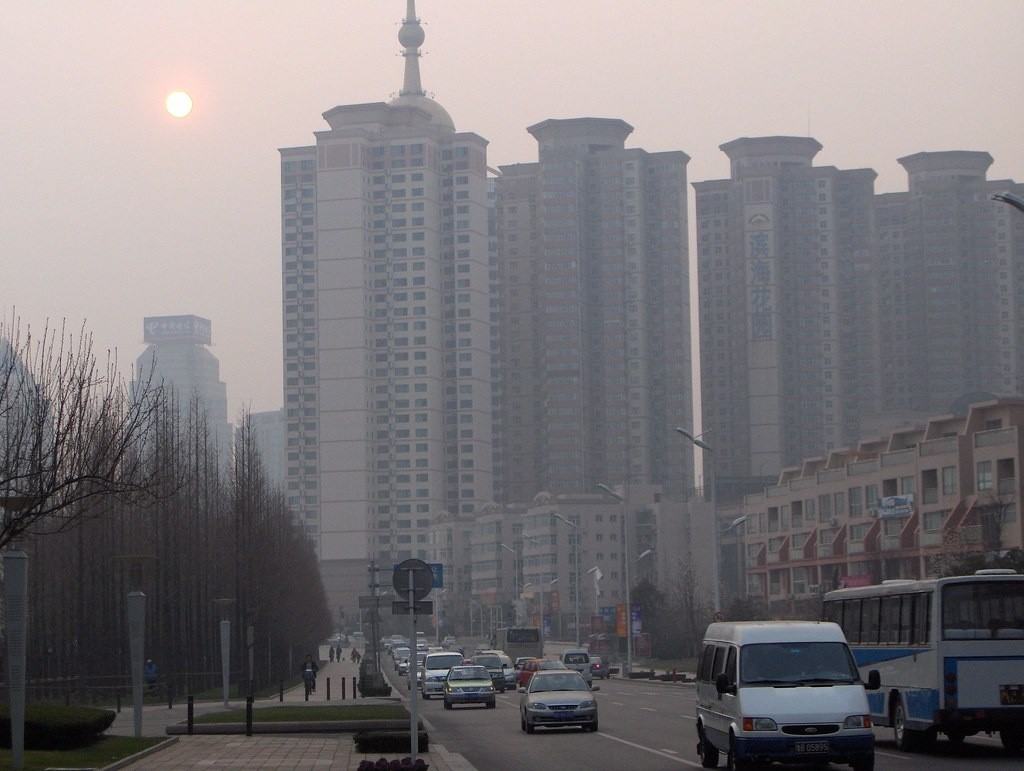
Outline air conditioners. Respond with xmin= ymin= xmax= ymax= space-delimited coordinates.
xmin=867 ymin=508 xmax=877 ymax=517
xmin=829 ymin=518 xmax=836 ymax=525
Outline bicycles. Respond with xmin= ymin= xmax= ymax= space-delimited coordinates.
xmin=299 ymin=663 xmax=318 ymax=701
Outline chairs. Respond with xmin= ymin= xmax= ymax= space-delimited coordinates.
xmin=855 ymin=618 xmax=1024 ymax=648
xmin=536 ymin=677 xmax=549 ymax=689
xmin=453 ymin=671 xmax=461 ymax=678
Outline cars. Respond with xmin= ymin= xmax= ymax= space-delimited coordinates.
xmin=515 ymin=656 xmax=537 ymax=671
xmin=441 ymin=665 xmax=496 ymax=709
xmin=519 ymin=659 xmax=571 ymax=686
xmin=517 ymin=670 xmax=601 ymax=733
xmin=384 ymin=631 xmax=504 ymax=690
xmin=589 ymin=657 xmax=611 ymax=679
xmin=499 ymin=654 xmax=517 ymax=690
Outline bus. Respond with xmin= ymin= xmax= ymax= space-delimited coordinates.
xmin=820 ymin=569 xmax=1024 ymax=759
xmin=490 ymin=625 xmax=544 ymax=663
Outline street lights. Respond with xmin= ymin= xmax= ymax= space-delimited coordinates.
xmin=675 ymin=426 xmax=716 ymax=616
xmin=598 ymin=482 xmax=634 ymax=673
xmin=521 ymin=533 xmax=545 ymax=653
xmin=554 ymin=512 xmax=581 ymax=649
xmin=499 ymin=543 xmax=518 ymax=626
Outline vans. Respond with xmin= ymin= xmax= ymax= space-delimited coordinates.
xmin=470 ymin=654 xmax=508 ymax=693
xmin=555 ymin=648 xmax=594 ymax=687
xmin=692 ymin=619 xmax=882 ymax=771
xmin=417 ymin=651 xmax=466 ymax=700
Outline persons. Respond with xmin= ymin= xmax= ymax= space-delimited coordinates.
xmin=563 ymin=675 xmax=579 ymax=689
xmin=329 ymin=646 xmax=335 ymax=662
xmin=301 ymin=655 xmax=318 ymax=689
xmin=143 ymin=659 xmax=159 ymax=696
xmin=536 ymin=677 xmax=551 ymax=691
xmin=336 ymin=645 xmax=342 ymax=663
xmin=351 ymin=647 xmax=361 ymax=663
